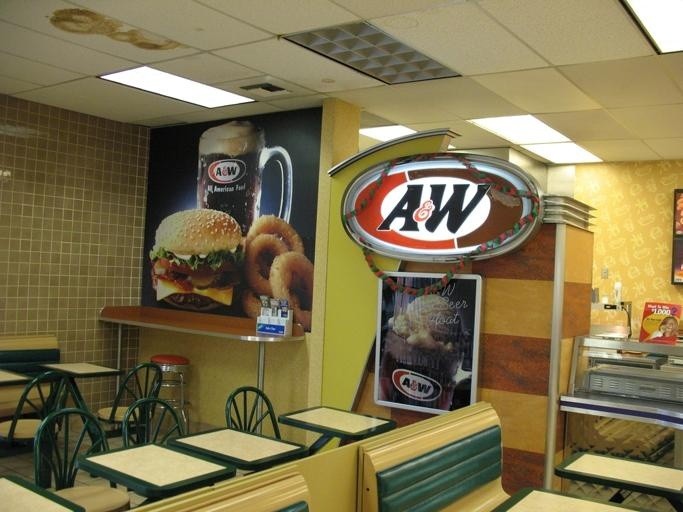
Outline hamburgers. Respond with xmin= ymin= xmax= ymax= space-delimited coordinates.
xmin=150 ymin=207 xmax=244 ymax=314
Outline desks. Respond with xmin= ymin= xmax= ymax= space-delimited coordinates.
xmin=98 ymin=304 xmax=306 ymax=436
xmin=0 ymin=363 xmax=683 ymax=512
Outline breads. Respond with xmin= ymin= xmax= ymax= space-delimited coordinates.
xmin=406 ymin=293 xmax=463 ymax=343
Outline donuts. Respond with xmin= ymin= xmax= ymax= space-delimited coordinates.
xmin=239 ymin=214 xmax=312 ymax=334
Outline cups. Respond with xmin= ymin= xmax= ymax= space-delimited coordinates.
xmin=195 ymin=123 xmax=294 ymax=237
xmin=380 ymin=327 xmax=467 ymax=410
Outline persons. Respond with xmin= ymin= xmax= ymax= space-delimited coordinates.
xmin=651 ymin=317 xmax=678 ymax=340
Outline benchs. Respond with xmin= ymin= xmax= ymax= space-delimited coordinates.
xmin=0 ymin=334 xmax=61 ymax=419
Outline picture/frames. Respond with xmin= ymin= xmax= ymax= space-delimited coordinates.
xmin=671 ymin=189 xmax=683 ymax=285
xmin=373 ymin=271 xmax=483 ymax=416
